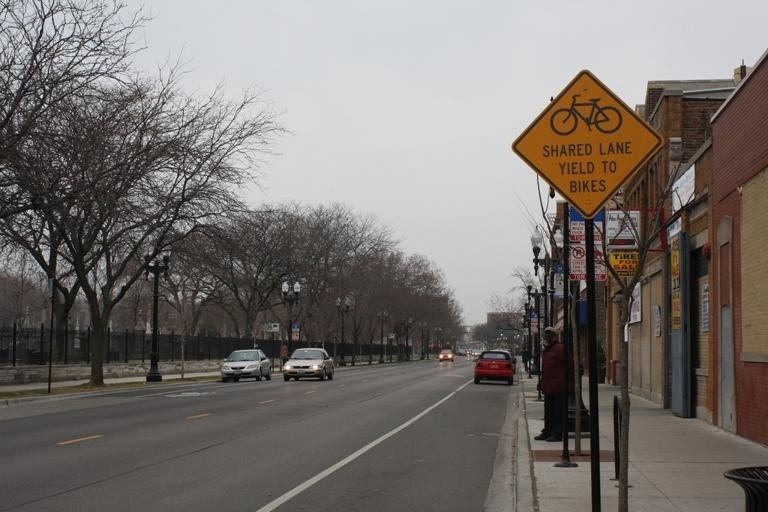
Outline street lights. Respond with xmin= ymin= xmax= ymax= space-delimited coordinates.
xmin=334 ymin=295 xmax=353 ymax=366
xmin=282 ymin=279 xmax=301 ymax=357
xmin=402 ymin=317 xmax=444 ymax=361
xmin=519 ymin=279 xmax=547 ymax=375
xmin=142 ymin=239 xmax=172 ymax=382
xmin=377 ymin=308 xmax=390 ymax=363
xmin=530 ymin=224 xmax=565 ymax=328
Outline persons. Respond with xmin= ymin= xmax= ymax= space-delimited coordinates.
xmin=535 ymin=326 xmax=568 ymax=441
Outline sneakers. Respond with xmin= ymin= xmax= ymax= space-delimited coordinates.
xmin=534 ymin=429 xmax=563 ymax=442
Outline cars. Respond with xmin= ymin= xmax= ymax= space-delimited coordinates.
xmin=220 ymin=349 xmax=273 ymax=382
xmin=439 ymin=348 xmax=454 ymax=361
xmin=473 ymin=350 xmax=513 ymax=385
xmin=281 ymin=348 xmax=335 ymax=382
xmin=498 ymin=348 xmax=517 ymax=373
xmin=456 ymin=348 xmax=482 ymax=362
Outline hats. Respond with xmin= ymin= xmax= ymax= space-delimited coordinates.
xmin=544 ymin=326 xmax=555 ymax=333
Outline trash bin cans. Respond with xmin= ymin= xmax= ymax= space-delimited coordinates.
xmin=723 ymin=465 xmax=768 ymax=512
